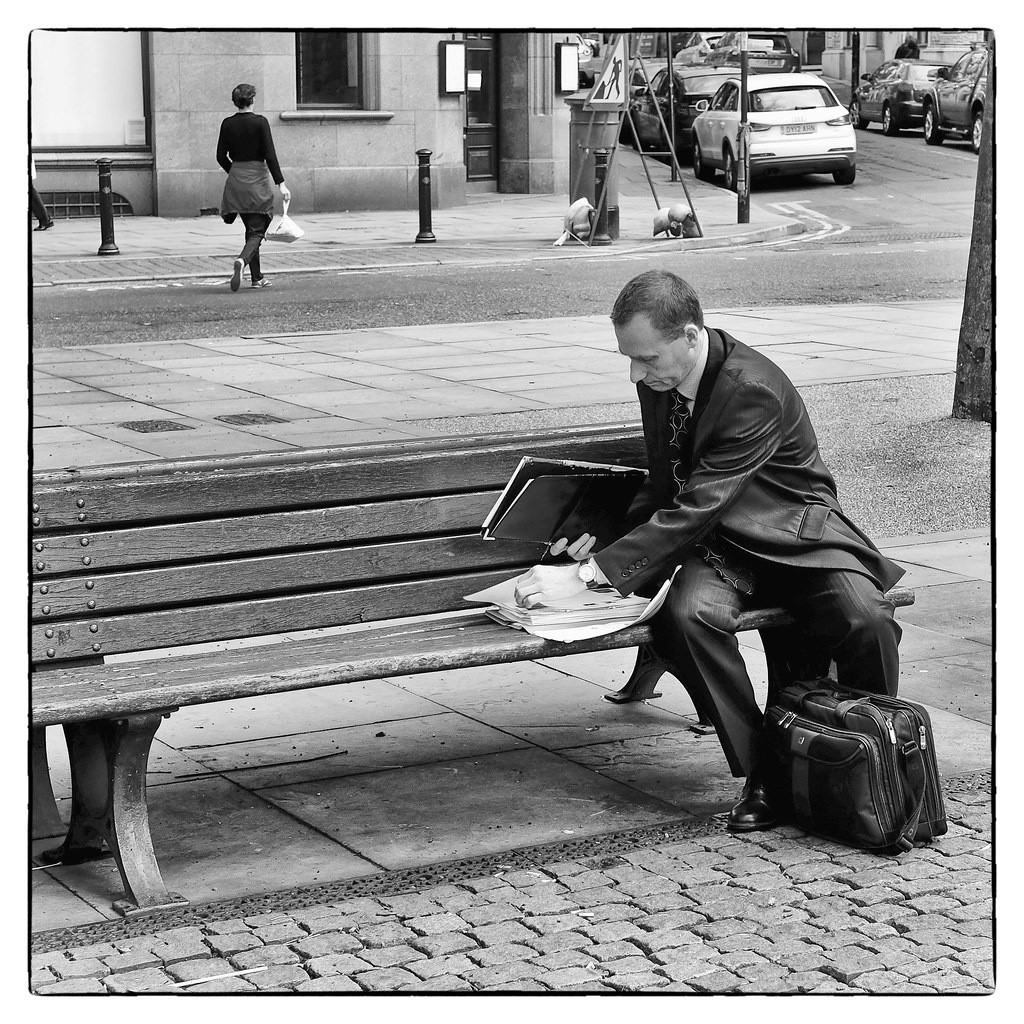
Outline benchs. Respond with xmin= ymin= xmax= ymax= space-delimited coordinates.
xmin=29 ymin=423 xmax=913 ymax=918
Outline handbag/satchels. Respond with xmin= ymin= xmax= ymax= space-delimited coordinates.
xmin=763 ymin=679 xmax=946 ymax=853
xmin=264 ymin=198 xmax=303 ymax=243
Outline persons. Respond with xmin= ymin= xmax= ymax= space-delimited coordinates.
xmin=31 ymin=154 xmax=54 ymax=231
xmin=894 ymin=33 xmax=920 ymax=59
xmin=513 ymin=268 xmax=906 ymax=834
xmin=216 ymin=83 xmax=292 ymax=293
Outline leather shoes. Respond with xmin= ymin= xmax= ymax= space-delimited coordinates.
xmin=727 ymin=772 xmax=781 ymax=831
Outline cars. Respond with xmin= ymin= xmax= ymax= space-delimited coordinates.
xmin=575 ymin=32 xmax=803 ymax=143
xmin=690 ymin=71 xmax=858 ymax=193
xmin=634 ymin=62 xmax=759 ymax=166
xmin=847 ymin=57 xmax=957 ymax=137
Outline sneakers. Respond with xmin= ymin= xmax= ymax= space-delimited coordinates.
xmin=230 ymin=258 xmax=245 ymax=291
xmin=251 ymin=275 xmax=272 ymax=289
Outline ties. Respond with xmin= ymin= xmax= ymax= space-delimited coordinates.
xmin=668 ymin=389 xmax=758 ymax=597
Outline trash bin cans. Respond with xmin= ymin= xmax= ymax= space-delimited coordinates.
xmin=563 ymin=93 xmax=625 ymax=240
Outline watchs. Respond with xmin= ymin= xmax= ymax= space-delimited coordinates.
xmin=577 ymin=559 xmax=599 ymax=589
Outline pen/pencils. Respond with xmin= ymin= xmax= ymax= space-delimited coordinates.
xmin=540 ymin=544 xmax=551 ymax=562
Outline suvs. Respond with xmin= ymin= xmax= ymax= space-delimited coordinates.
xmin=922 ymin=41 xmax=989 ymax=154
